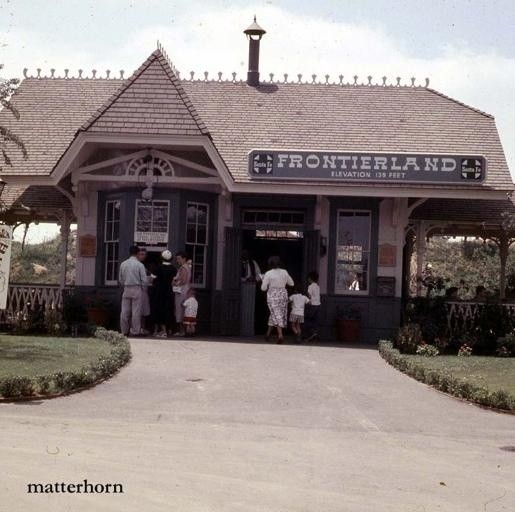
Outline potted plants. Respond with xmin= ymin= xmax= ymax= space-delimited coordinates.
xmin=393 ymin=322 xmax=424 ymax=355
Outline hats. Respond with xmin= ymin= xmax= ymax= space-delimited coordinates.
xmin=161 ymin=250 xmax=174 ymax=263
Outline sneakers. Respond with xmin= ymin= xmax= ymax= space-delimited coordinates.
xmin=152 ymin=330 xmax=168 ymax=339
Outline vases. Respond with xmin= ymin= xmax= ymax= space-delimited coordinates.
xmin=85 ymin=307 xmax=114 ymax=336
xmin=337 ymin=311 xmax=364 ymax=343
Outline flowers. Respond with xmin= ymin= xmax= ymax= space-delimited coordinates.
xmin=335 ymin=302 xmax=367 ymax=318
xmin=83 ymin=290 xmax=116 ymax=312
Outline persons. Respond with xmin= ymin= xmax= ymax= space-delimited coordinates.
xmin=240 ymin=245 xmax=260 ymax=283
xmin=306 ymin=274 xmax=324 ymax=341
xmin=284 ymin=285 xmax=312 ymax=341
xmin=117 ymin=245 xmax=199 ymax=338
xmin=259 ymin=255 xmax=295 ymax=343
